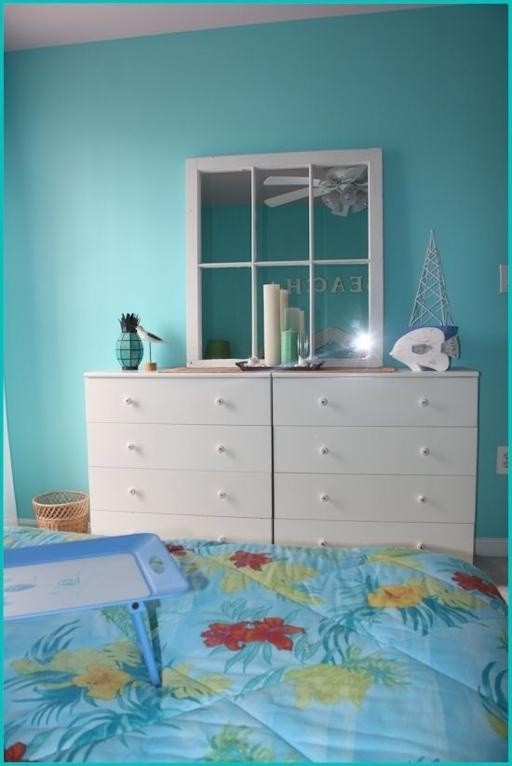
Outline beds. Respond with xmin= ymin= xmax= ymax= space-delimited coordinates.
xmin=4 ymin=528 xmax=508 ymax=761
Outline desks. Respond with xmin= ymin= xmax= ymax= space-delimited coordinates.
xmin=5 ymin=533 xmax=190 ymax=687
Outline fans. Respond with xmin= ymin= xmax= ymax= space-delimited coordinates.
xmin=263 ymin=163 xmax=366 ymax=208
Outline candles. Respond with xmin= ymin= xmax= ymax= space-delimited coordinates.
xmin=281 ymin=330 xmax=297 ymax=366
xmin=277 ymin=290 xmax=289 ymax=364
xmin=262 ymin=283 xmax=281 ymax=366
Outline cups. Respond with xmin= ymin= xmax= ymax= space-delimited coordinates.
xmin=206 ymin=339 xmax=231 ymax=358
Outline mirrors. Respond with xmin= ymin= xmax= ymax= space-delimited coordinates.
xmin=185 ymin=148 xmax=383 ymax=368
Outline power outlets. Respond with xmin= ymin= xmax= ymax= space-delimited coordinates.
xmin=496 ymin=445 xmax=509 ymax=475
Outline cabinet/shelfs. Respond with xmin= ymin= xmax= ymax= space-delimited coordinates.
xmin=84 ymin=367 xmax=480 ymax=567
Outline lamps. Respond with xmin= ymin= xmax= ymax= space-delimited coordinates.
xmin=322 ymin=177 xmax=368 ymax=216
xmin=115 ymin=312 xmax=143 ymax=371
